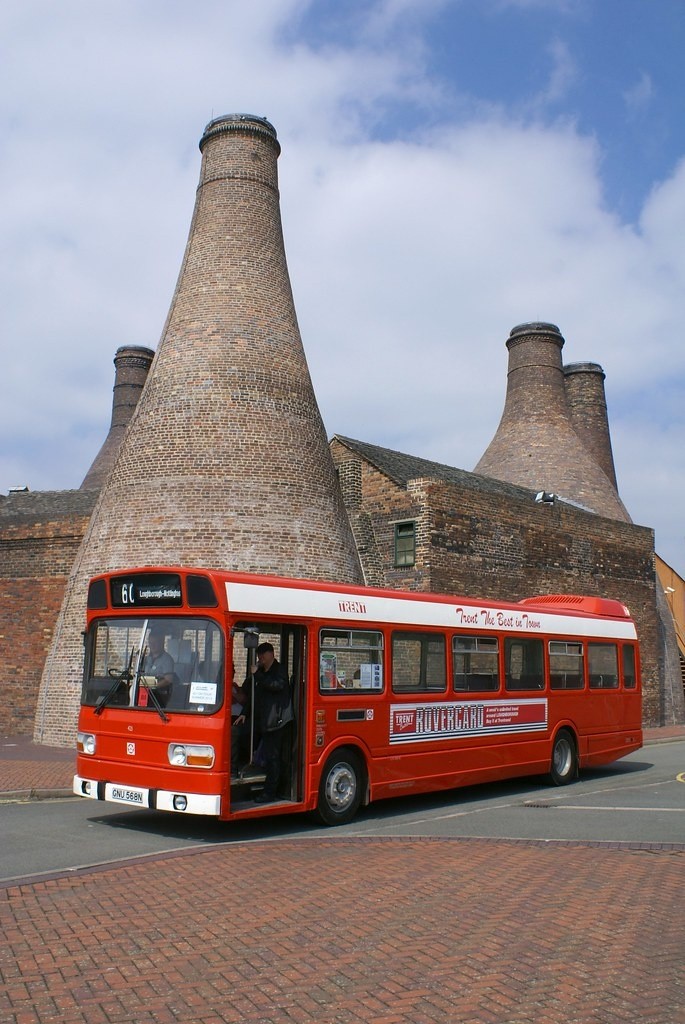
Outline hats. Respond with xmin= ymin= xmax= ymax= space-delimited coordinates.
xmin=256 ymin=642 xmax=274 ymax=654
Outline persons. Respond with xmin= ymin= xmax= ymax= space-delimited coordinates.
xmin=232 ymin=643 xmax=292 ymax=803
xmin=139 ymin=630 xmax=174 ymax=708
xmin=187 ymin=661 xmax=219 ymax=711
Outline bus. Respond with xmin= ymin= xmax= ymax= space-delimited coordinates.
xmin=71 ymin=564 xmax=643 ymax=825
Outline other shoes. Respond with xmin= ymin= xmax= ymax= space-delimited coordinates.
xmin=230 ymin=769 xmax=241 ymax=780
xmin=255 ymin=793 xmax=275 ymax=802
